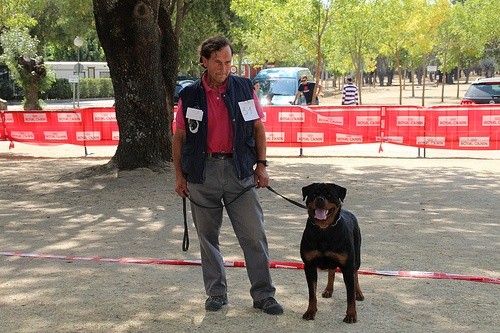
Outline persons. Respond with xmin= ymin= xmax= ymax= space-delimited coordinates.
xmin=289 ymin=75 xmax=322 ymax=106
xmin=171 ymin=35 xmax=283 ymax=316
xmin=253 ymin=81 xmax=264 ymax=105
xmin=341 ymin=77 xmax=359 ymax=105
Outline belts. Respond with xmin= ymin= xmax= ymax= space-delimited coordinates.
xmin=205 ymin=151 xmax=234 ymax=160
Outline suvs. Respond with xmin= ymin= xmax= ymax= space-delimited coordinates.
xmin=461 ymin=78 xmax=500 ymax=106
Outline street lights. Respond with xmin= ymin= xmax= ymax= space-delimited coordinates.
xmin=74 ymin=36 xmax=84 ymax=108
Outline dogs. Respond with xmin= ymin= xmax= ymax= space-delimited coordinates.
xmin=299 ymin=182 xmax=365 ymax=324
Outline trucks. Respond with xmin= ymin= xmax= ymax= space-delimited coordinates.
xmin=43 ymin=62 xmax=113 ymax=97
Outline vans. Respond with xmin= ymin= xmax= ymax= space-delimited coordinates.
xmin=251 ymin=67 xmax=316 ymax=107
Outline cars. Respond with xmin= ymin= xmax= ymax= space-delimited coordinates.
xmin=173 ymin=79 xmax=196 ymax=105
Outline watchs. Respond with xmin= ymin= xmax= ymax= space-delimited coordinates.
xmin=257 ymin=159 xmax=270 ymax=167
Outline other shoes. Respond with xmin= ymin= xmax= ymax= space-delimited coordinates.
xmin=204 ymin=293 xmax=228 ymax=310
xmin=252 ymin=296 xmax=283 ymax=315
